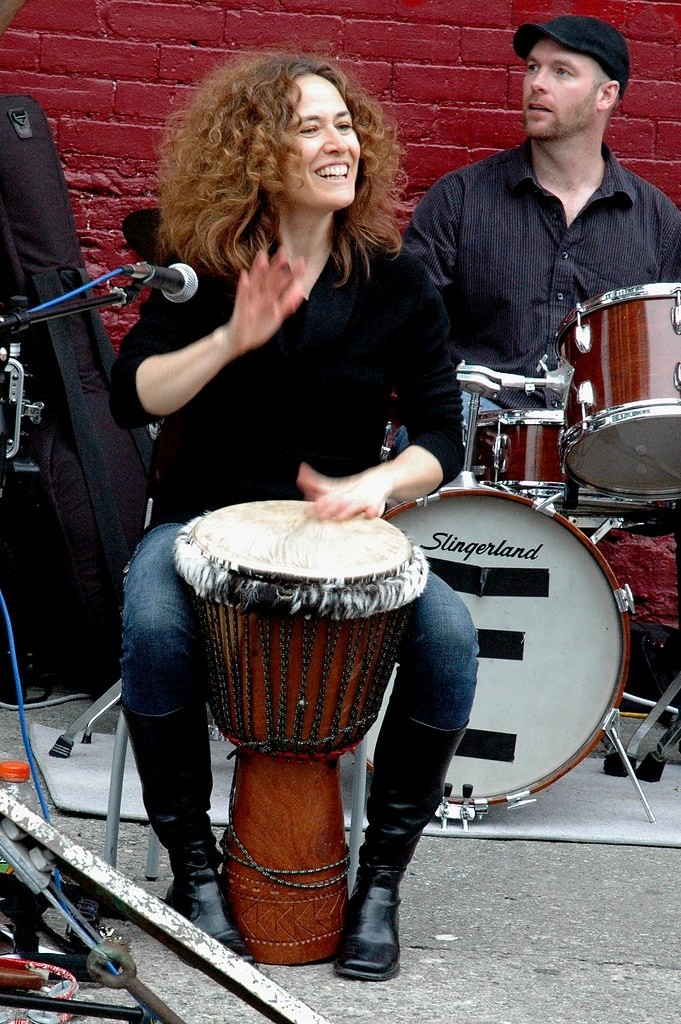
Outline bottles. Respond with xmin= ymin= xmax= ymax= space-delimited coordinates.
xmin=0 ymin=761 xmax=39 ymax=876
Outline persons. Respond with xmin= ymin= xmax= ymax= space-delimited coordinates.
xmin=399 ymin=16 xmax=681 ymax=432
xmin=108 ymin=54 xmax=480 ymax=982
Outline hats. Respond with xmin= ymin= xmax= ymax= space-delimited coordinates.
xmin=513 ymin=15 xmax=629 ymax=97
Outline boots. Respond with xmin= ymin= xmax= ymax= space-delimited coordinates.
xmin=333 ymin=704 xmax=469 ymax=980
xmin=120 ymin=693 xmax=256 ymax=965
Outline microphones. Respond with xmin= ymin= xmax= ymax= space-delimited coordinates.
xmin=122 ymin=263 xmax=200 ymax=302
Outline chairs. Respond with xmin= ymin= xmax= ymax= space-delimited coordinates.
xmin=101 ymin=435 xmax=366 ymax=900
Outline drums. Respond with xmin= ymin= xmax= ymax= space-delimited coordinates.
xmin=551 ymin=280 xmax=681 ymax=500
xmin=468 ymin=405 xmax=574 ymax=517
xmin=348 ymin=484 xmax=632 ymax=818
xmin=174 ymin=499 xmax=432 ymax=967
